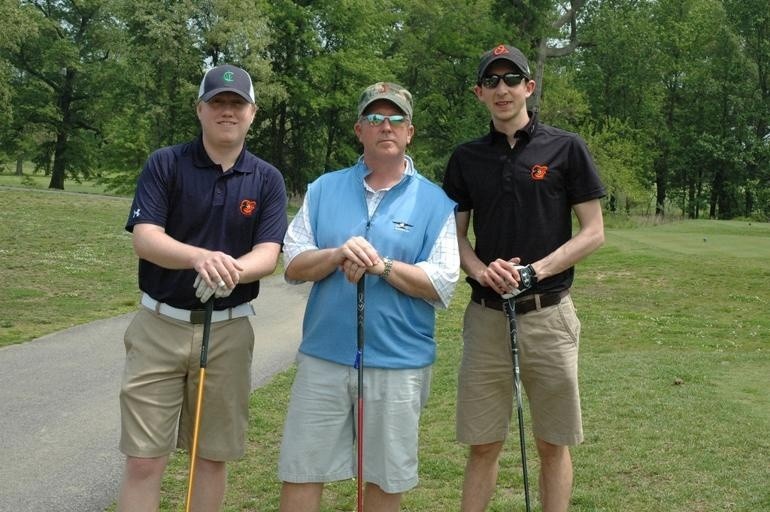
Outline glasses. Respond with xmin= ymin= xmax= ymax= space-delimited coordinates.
xmin=362 ymin=114 xmax=410 ymax=127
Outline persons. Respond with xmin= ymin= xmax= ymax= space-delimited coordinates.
xmin=442 ymin=44 xmax=608 ymax=512
xmin=276 ymin=81 xmax=461 ymax=512
xmin=118 ymin=64 xmax=289 ymax=512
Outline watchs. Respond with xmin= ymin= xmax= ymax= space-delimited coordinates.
xmin=378 ymin=258 xmax=395 ymax=283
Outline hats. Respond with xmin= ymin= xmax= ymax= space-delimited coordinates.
xmin=480 ymin=72 xmax=526 ymax=90
xmin=478 ymin=45 xmax=531 ymax=85
xmin=357 ymin=82 xmax=414 ymax=127
xmin=198 ymin=65 xmax=256 ymax=105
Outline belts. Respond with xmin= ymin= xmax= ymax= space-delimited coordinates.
xmin=142 ymin=292 xmax=256 ymax=323
xmin=470 ymin=288 xmax=570 ymax=317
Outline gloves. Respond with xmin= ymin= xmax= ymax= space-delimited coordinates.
xmin=497 ymin=263 xmax=539 ymax=300
